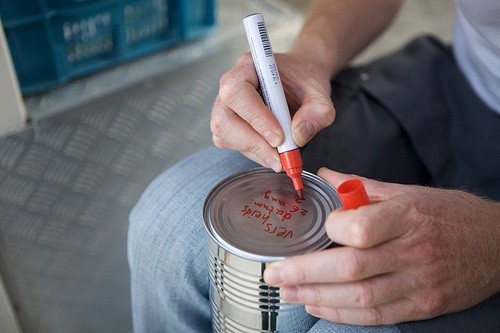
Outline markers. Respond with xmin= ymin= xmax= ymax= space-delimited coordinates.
xmin=241 ymin=12 xmax=306 ymax=201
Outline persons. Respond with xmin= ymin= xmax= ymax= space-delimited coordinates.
xmin=127 ymin=0 xmax=500 ymax=333
